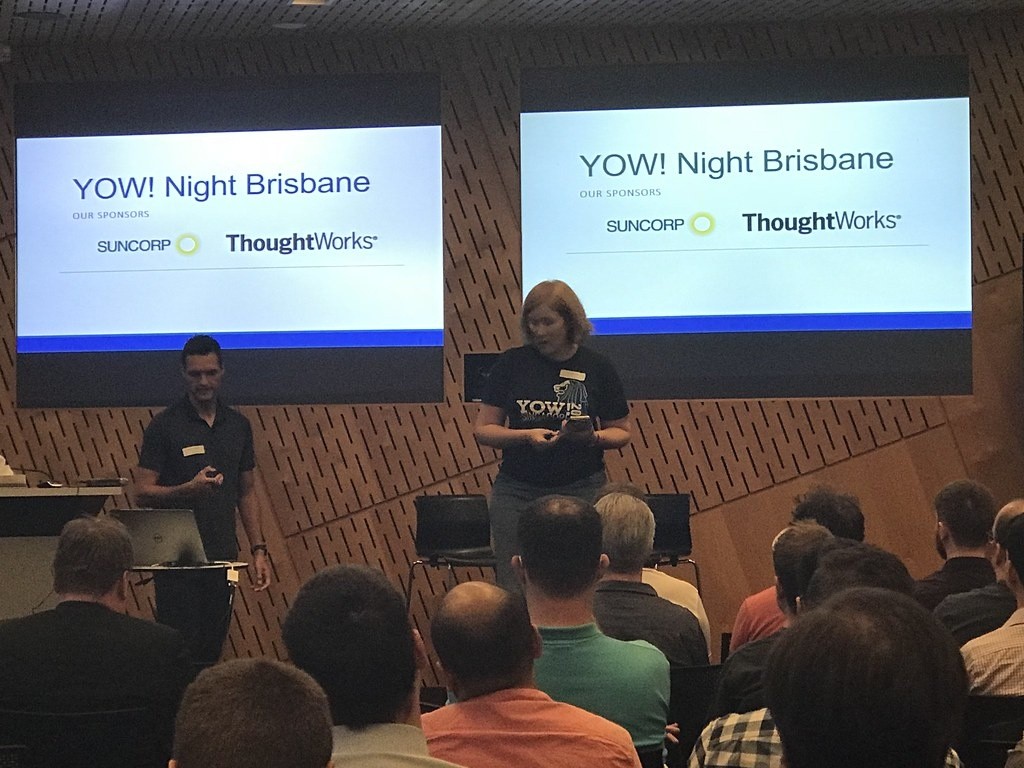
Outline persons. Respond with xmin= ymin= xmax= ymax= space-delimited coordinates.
xmin=591 ymin=482 xmax=713 ymax=668
xmin=420 ymin=581 xmax=642 ymax=768
xmin=0 ymin=516 xmax=196 ymax=768
xmin=279 ymin=562 xmax=470 ymax=768
xmin=472 ymin=279 xmax=632 ymax=593
xmin=683 ymin=479 xmax=1024 ymax=768
xmin=509 ymin=493 xmax=681 ymax=768
xmin=168 ymin=657 xmax=335 ymax=768
xmin=134 ymin=335 xmax=272 ymax=671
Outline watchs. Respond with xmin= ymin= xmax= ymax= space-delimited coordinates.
xmin=251 ymin=543 xmax=267 ymax=555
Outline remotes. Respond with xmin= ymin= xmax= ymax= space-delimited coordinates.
xmin=86 ymin=477 xmax=130 ymax=487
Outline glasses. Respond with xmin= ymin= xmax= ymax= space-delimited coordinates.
xmin=986 ymin=529 xmax=1002 ymax=548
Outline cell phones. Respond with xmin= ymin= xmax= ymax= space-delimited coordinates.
xmin=565 ymin=416 xmax=590 ymax=429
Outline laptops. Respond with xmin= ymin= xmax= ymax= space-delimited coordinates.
xmin=109 ymin=509 xmax=236 ymax=566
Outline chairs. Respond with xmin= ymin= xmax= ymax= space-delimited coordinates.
xmin=641 ymin=494 xmax=701 ymax=600
xmin=405 ymin=495 xmax=502 ymax=625
xmin=417 ymin=662 xmax=1024 ymax=768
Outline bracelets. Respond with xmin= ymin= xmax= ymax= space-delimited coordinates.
xmin=596 ymin=434 xmax=600 ymax=447
